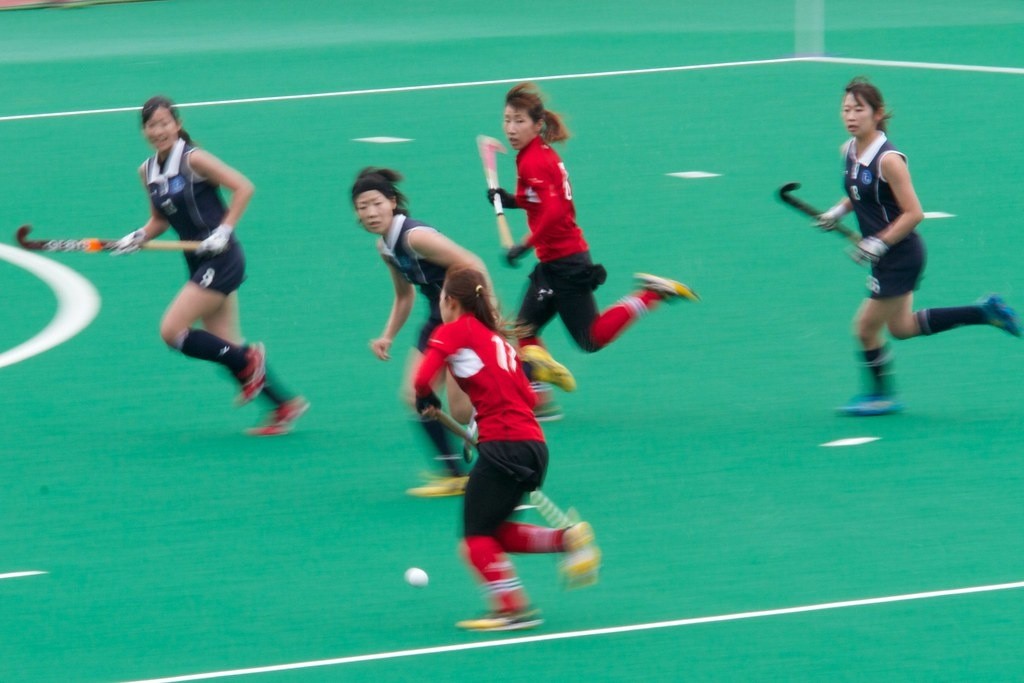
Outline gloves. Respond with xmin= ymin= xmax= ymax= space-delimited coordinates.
xmin=506 ymin=245 xmax=526 ymax=266
xmin=116 ymin=229 xmax=150 ymax=254
xmin=488 ymin=187 xmax=518 ymax=207
xmin=197 ymin=227 xmax=230 ymax=255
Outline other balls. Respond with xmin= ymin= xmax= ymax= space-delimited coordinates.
xmin=403 ymin=567 xmax=431 ymax=588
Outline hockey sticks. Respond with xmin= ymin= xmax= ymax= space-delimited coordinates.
xmin=16 ymin=221 xmax=207 ymax=254
xmin=474 ymin=132 xmax=515 ymax=252
xmin=776 ymin=181 xmax=868 ymax=246
xmin=462 ymin=404 xmax=479 ymax=464
xmin=433 ymin=406 xmax=579 ymax=532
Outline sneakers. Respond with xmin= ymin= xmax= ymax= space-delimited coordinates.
xmin=459 ymin=607 xmax=543 ymax=632
xmin=637 ymin=272 xmax=697 ymax=301
xmin=256 ymin=397 xmax=309 ymax=434
xmin=241 ymin=342 xmax=267 ymax=400
xmin=522 ymin=345 xmax=574 ymax=391
xmin=565 ymin=525 xmax=596 ymax=580
xmin=411 ymin=476 xmax=467 ymax=497
xmin=983 ymin=295 xmax=1023 ymax=336
xmin=845 ymin=393 xmax=906 ymax=414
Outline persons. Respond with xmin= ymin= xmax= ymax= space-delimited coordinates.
xmin=110 ymin=95 xmax=311 ymax=435
xmin=488 ymin=83 xmax=701 ymax=422
xmin=350 ymin=165 xmax=600 ymax=631
xmin=812 ymin=74 xmax=1024 ymax=416
